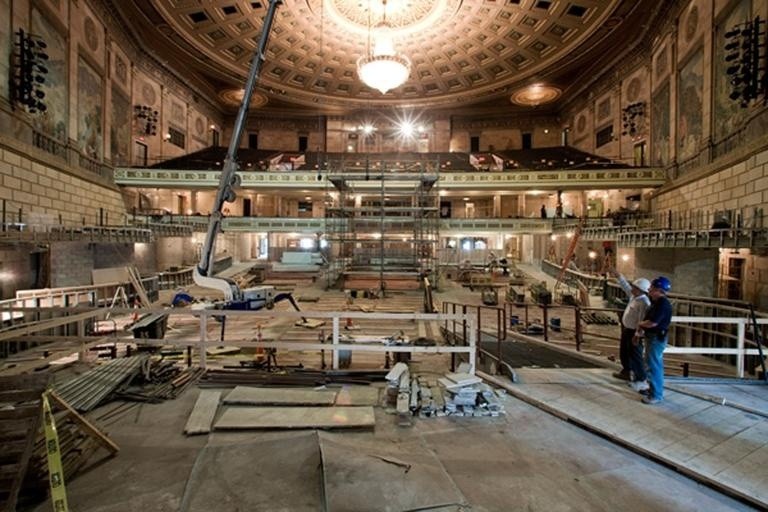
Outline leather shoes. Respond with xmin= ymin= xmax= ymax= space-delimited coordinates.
xmin=612 ymin=370 xmax=664 ymax=405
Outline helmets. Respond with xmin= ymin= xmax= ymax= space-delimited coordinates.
xmin=631 ymin=276 xmax=672 ymax=294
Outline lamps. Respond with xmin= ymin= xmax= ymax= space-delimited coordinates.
xmin=357 ymin=0 xmax=412 ymax=97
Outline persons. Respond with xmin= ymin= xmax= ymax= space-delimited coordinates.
xmin=640 ymin=276 xmax=674 ymax=403
xmin=608 ymin=265 xmax=651 ymax=382
